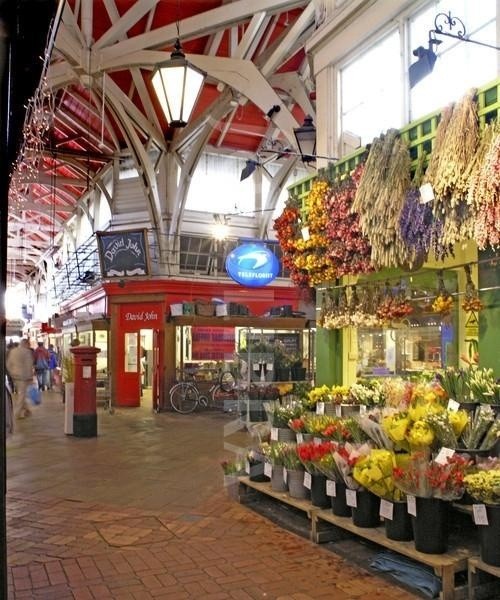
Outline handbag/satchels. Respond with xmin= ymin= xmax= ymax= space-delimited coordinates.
xmin=53 ymin=370 xmax=62 ymax=386
xmin=30 ymin=385 xmax=41 ymax=404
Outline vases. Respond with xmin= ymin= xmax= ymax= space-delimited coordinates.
xmin=332 ymin=481 xmax=500 ymax=567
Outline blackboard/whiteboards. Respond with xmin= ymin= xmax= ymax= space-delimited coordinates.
xmin=95 ymin=229 xmax=152 ymax=279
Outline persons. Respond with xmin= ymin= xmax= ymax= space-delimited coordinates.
xmin=5 ymin=336 xmax=63 ymax=436
xmin=139 ymin=346 xmax=147 ymax=397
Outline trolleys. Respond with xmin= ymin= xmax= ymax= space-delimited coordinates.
xmin=93 ymin=369 xmax=116 ymax=416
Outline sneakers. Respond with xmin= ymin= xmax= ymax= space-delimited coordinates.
xmin=39 ymin=384 xmax=54 ymax=392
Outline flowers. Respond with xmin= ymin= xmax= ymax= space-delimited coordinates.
xmin=315 ymin=264 xmax=484 ymax=331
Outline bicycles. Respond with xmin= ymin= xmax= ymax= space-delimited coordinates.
xmin=168 ymin=366 xmax=239 ymax=414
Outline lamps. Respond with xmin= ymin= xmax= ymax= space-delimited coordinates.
xmin=149 ymin=18 xmax=208 ymax=128
xmin=240 ymin=146 xmax=289 ymax=182
xmin=408 ymin=29 xmax=500 ymax=89
